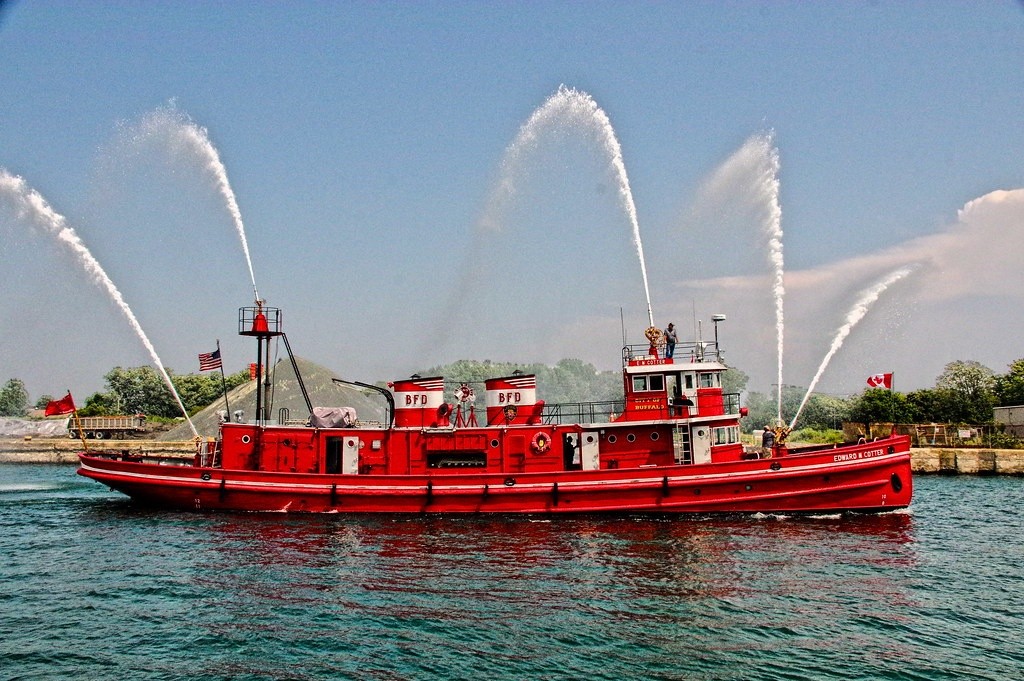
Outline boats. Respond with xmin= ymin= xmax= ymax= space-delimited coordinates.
xmin=42 ymin=299 xmax=918 ymax=515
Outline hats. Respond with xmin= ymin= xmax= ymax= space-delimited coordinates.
xmin=669 ymin=322 xmax=675 ymax=325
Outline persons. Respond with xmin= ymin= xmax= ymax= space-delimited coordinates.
xmin=775 ymin=423 xmax=786 ymax=458
xmin=663 ymin=323 xmax=680 ymax=358
xmin=564 ymin=435 xmax=579 ymax=469
xmin=760 ymin=425 xmax=780 ymax=459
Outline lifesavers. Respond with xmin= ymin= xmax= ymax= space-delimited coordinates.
xmin=532 ymin=432 xmax=551 ymax=453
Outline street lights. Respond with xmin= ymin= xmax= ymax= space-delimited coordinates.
xmin=709 ymin=312 xmax=727 ymax=384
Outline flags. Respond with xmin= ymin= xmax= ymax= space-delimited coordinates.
xmin=45 ymin=394 xmax=76 ymax=415
xmin=199 ymin=349 xmax=223 ymax=370
xmin=865 ymin=373 xmax=893 ymax=390
